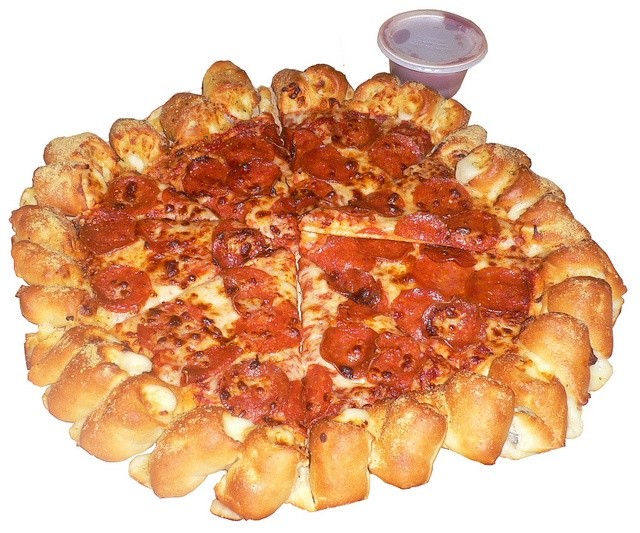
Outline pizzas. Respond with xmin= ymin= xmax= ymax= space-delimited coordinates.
xmin=10 ymin=59 xmax=627 ymax=522
